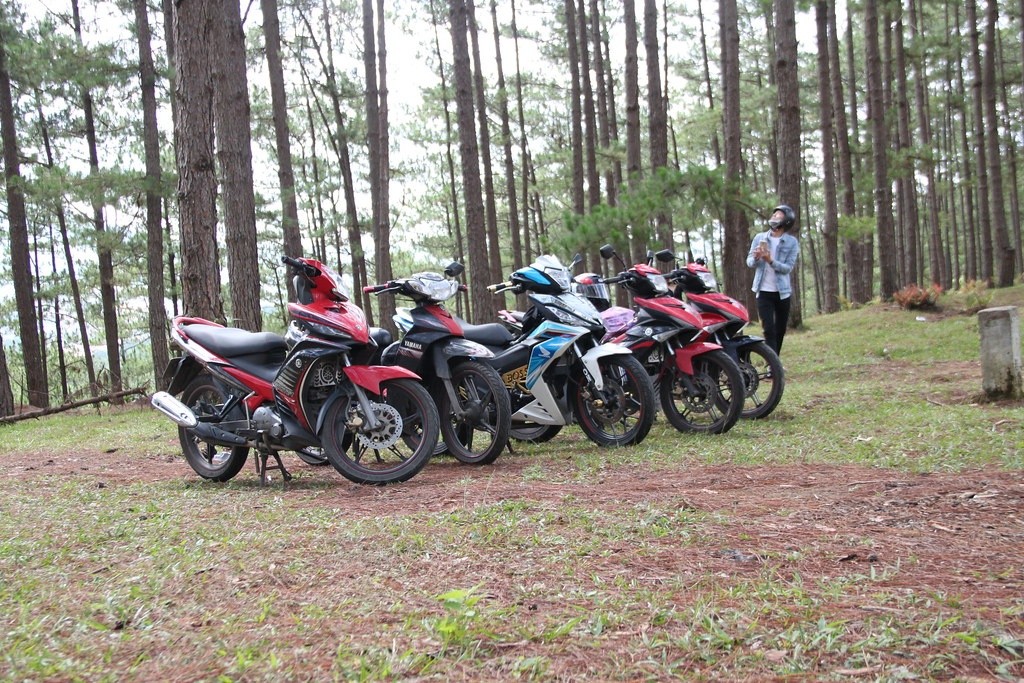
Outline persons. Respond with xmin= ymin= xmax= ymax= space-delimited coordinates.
xmin=747 ymin=206 xmax=799 ymax=378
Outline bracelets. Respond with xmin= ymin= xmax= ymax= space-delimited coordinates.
xmin=769 ymin=260 xmax=773 ymax=264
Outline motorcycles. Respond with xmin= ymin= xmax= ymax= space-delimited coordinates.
xmin=497 ymin=244 xmax=746 ymax=444
xmin=391 ymin=253 xmax=656 ymax=458
xmin=571 ymin=249 xmax=785 ymax=420
xmin=295 ymin=261 xmax=511 ymax=467
xmin=151 ymin=255 xmax=440 ymax=486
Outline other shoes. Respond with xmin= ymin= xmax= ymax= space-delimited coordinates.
xmin=766 ymin=368 xmax=772 ymax=377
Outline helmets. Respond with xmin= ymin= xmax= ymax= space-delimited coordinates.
xmin=572 ymin=272 xmax=612 ymax=312
xmin=768 ymin=204 xmax=795 ymax=231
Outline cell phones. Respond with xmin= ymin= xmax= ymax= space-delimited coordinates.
xmin=760 ymin=240 xmax=767 ymax=251
